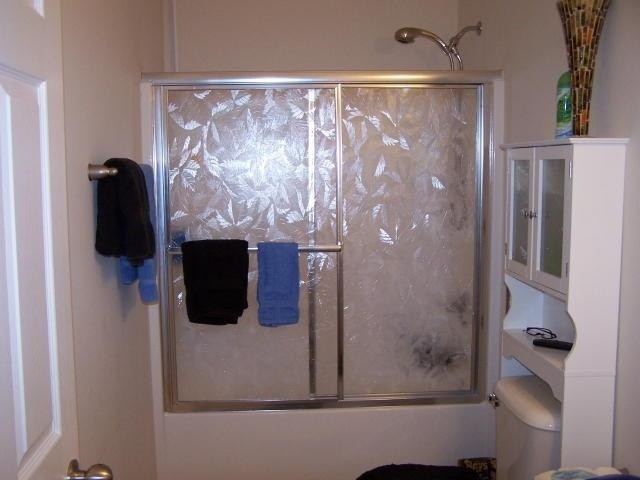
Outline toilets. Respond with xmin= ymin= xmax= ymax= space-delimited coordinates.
xmin=488 ymin=375 xmax=562 ymax=480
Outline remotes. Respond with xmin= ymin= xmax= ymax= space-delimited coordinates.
xmin=533 ymin=339 xmax=573 ymax=350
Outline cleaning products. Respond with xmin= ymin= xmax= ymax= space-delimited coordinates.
xmin=555 ymin=70 xmax=573 ymax=139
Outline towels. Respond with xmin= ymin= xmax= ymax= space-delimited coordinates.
xmin=256 ymin=242 xmax=300 ymax=326
xmin=94 ymin=156 xmax=160 ymax=304
xmin=179 ymin=239 xmax=249 ymax=325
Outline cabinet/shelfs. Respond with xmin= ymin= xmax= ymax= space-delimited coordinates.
xmin=503 ymin=145 xmax=572 ymax=297
